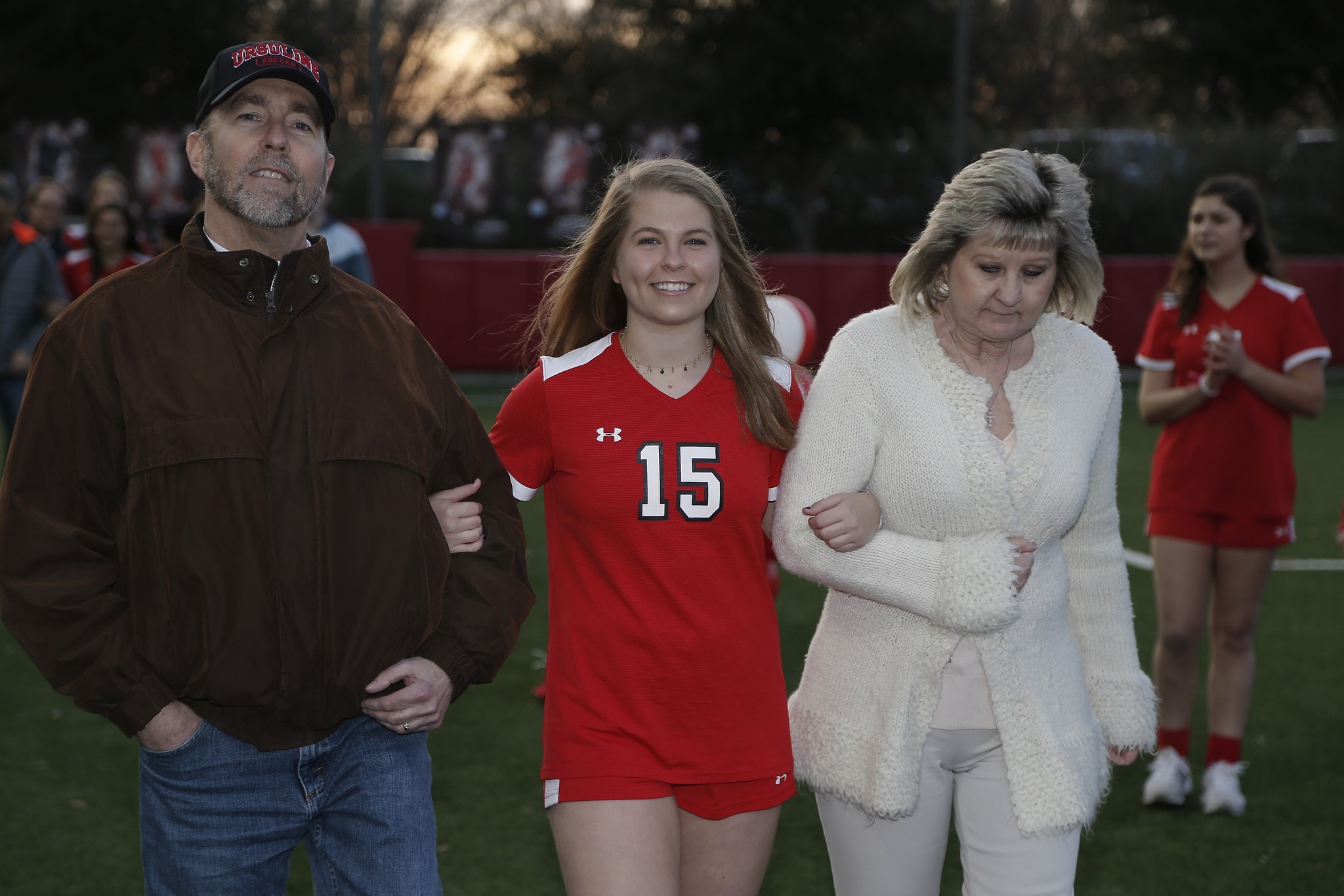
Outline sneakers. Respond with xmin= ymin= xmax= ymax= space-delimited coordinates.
xmin=1200 ymin=759 xmax=1247 ymax=816
xmin=1141 ymin=746 xmax=1194 ymax=806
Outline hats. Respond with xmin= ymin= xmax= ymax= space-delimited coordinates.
xmin=194 ymin=40 xmax=336 ymax=144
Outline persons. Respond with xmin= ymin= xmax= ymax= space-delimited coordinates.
xmin=772 ymin=149 xmax=1158 ymax=896
xmin=429 ymin=160 xmax=883 ymax=896
xmin=2 ymin=39 xmax=533 ymax=896
xmin=1135 ymin=175 xmax=1332 ymax=815
xmin=2 ymin=108 xmax=373 ymax=465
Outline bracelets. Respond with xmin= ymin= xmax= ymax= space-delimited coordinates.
xmin=1199 ymin=375 xmax=1217 ymax=397
xmin=858 ymin=488 xmax=882 ymax=530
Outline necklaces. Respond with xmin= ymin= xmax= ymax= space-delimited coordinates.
xmin=940 ymin=299 xmax=1013 ymax=427
xmin=621 ymin=326 xmax=708 ymax=375
